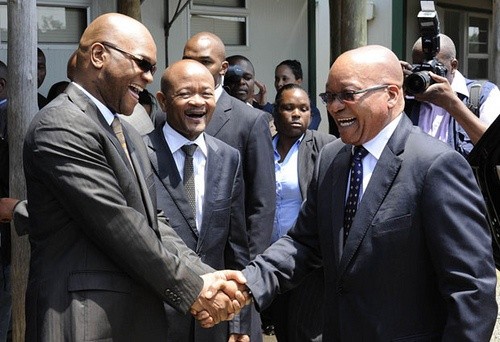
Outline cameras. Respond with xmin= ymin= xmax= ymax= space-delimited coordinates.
xmin=404 ymin=1 xmax=448 ymax=95
xmin=222 ymin=65 xmax=244 ymax=94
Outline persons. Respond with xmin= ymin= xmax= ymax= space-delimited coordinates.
xmin=189 ymin=43 xmax=499 ymax=342
xmin=0 ymin=31 xmax=500 ymax=342
xmin=22 ymin=11 xmax=255 ymax=342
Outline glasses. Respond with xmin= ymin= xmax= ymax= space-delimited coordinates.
xmin=100 ymin=42 xmax=157 ymax=76
xmin=319 ymin=85 xmax=389 ymax=104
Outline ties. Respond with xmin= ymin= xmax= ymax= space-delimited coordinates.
xmin=342 ymin=145 xmax=368 ymax=247
xmin=179 ymin=144 xmax=198 ymax=233
xmin=111 ymin=116 xmax=134 ymax=177
